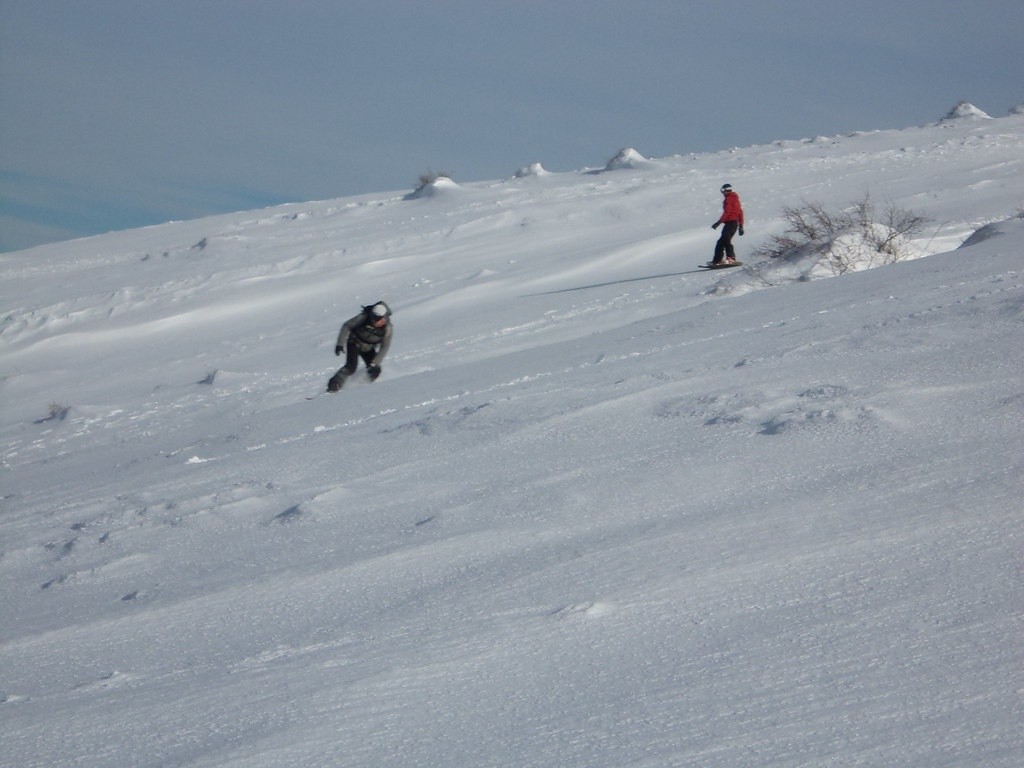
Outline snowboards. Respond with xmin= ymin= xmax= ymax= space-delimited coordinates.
xmin=696 ymin=261 xmax=743 ymax=269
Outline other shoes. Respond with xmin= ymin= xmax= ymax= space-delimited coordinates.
xmin=724 ymin=256 xmax=735 ymax=264
xmin=370 ymin=368 xmax=381 ymax=381
xmin=707 ymin=261 xmax=725 ymax=266
xmin=328 ymin=377 xmax=341 ymax=393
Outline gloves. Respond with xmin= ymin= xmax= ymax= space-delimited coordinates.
xmin=739 ymin=228 xmax=743 ymax=235
xmin=336 ymin=346 xmax=345 ymax=356
xmin=367 ymin=366 xmax=375 ymax=373
xmin=712 ymin=222 xmax=720 ymax=229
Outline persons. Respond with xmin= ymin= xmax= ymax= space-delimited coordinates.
xmin=706 ymin=184 xmax=743 ymax=265
xmin=327 ymin=301 xmax=393 ymax=392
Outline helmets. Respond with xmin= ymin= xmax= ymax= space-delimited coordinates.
xmin=369 ymin=304 xmax=389 ymax=319
xmin=721 ymin=184 xmax=732 ymax=192
